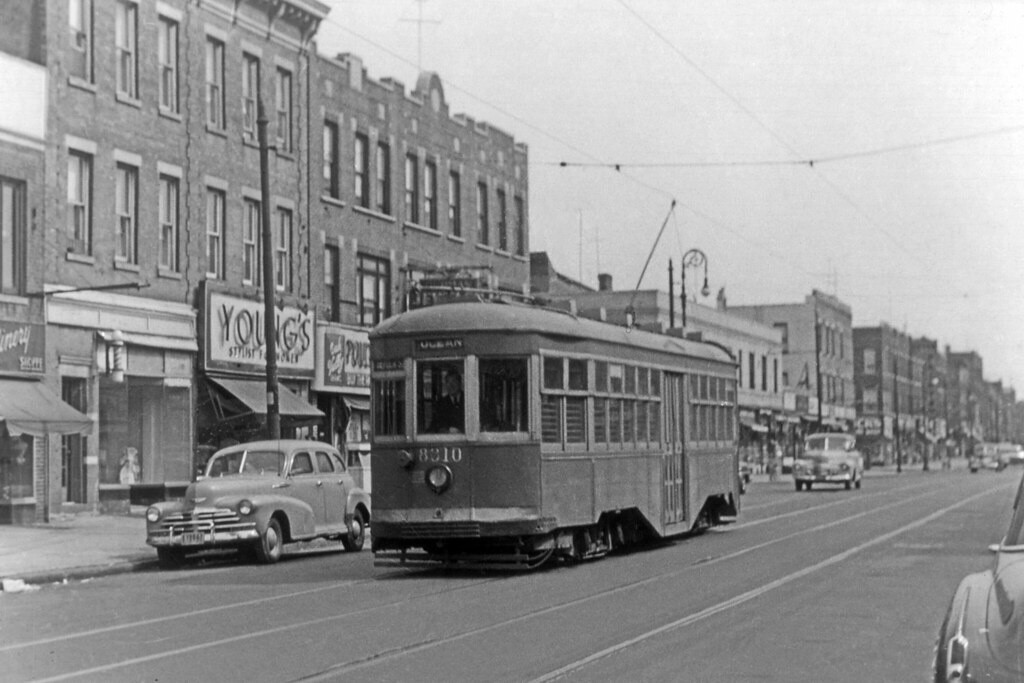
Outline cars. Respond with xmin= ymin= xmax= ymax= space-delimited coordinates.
xmin=933 ymin=475 xmax=1024 ymax=683
xmin=969 ymin=444 xmax=1004 ymax=473
xmin=792 ymin=433 xmax=864 ymax=492
xmin=146 ymin=440 xmax=372 ymax=568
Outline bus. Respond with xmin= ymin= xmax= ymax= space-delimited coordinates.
xmin=368 ymin=298 xmax=746 ymax=570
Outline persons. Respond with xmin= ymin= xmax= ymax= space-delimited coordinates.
xmin=425 ymin=371 xmax=495 ymax=434
xmin=768 ymin=438 xmax=783 ymax=481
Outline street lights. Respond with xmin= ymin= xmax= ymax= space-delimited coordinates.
xmin=680 ymin=249 xmax=712 ymax=327
xmin=922 ymin=355 xmax=939 ymax=471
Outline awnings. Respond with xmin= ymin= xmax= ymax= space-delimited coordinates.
xmin=803 ymin=415 xmax=837 ymax=426
xmin=206 ymin=372 xmax=326 ymax=422
xmin=739 ymin=415 xmax=769 ymax=433
xmin=0 ymin=371 xmax=95 ymax=437
